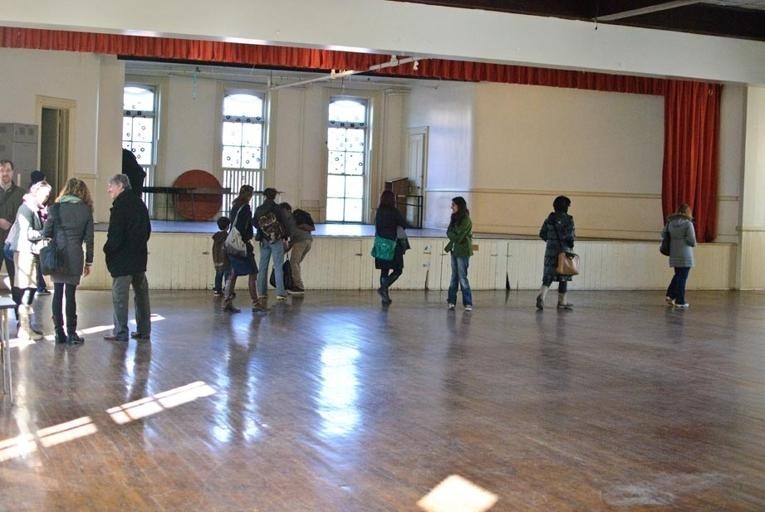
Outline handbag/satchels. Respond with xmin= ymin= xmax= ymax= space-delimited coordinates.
xmin=371 ymin=236 xmax=397 ymax=261
xmin=40 ymin=240 xmax=61 ymax=275
xmin=32 ymin=238 xmax=52 ymax=254
xmin=270 ymin=259 xmax=293 ymax=290
xmin=225 ymin=227 xmax=247 ymax=258
xmin=556 ymin=253 xmax=581 ymax=275
xmin=660 ymin=231 xmax=670 ymax=256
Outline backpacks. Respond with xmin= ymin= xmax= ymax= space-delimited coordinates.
xmin=256 ymin=205 xmax=285 ymax=244
xmin=292 ymin=208 xmax=316 ymax=231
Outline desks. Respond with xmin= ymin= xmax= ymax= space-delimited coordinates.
xmin=141 ymin=185 xmax=232 ymax=222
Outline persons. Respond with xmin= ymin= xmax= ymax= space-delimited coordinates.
xmin=371 ymin=190 xmax=410 ymax=305
xmin=211 ymin=183 xmax=316 ymax=313
xmin=443 ymin=196 xmax=473 ymax=312
xmin=534 ymin=196 xmax=580 ymax=311
xmin=659 ymin=201 xmax=697 ymax=310
xmin=1 ymin=160 xmax=94 ymax=346
xmin=101 ymin=172 xmax=154 ymax=341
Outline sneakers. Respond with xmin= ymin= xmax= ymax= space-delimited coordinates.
xmin=214 ymin=280 xmax=305 ymax=312
xmin=448 ymin=303 xmax=456 ymax=311
xmin=665 ymin=296 xmax=674 ymax=305
xmin=675 ymin=304 xmax=689 ymax=309
xmin=557 ymin=303 xmax=574 ymax=309
xmin=536 ymin=295 xmax=543 ymax=310
xmin=465 ymin=305 xmax=472 ymax=311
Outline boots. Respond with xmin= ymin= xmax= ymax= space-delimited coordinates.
xmin=377 ymin=272 xmax=398 ymax=305
xmin=16 ymin=289 xmax=149 ymax=344
